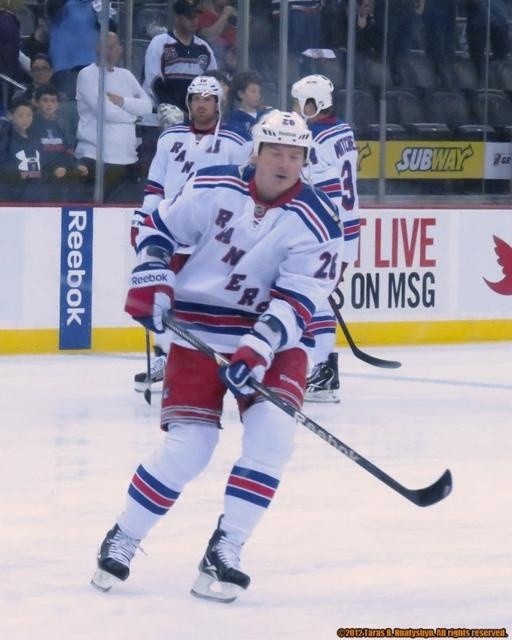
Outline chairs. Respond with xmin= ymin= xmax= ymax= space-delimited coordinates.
xmin=0 ymin=0 xmax=511 ymax=207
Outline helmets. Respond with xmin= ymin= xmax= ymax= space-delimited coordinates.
xmin=291 ymin=75 xmax=335 ymax=118
xmin=251 ymin=109 xmax=313 ymax=163
xmin=185 ymin=76 xmax=224 ymax=121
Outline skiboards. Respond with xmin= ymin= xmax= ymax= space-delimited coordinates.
xmin=161 ymin=315 xmax=451 ymax=506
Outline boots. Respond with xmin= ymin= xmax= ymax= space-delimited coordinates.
xmin=199 ymin=514 xmax=250 ymax=589
xmin=97 ymin=524 xmax=147 ymax=581
xmin=135 ymin=346 xmax=167 ymax=382
xmin=306 ymin=353 xmax=339 ymax=392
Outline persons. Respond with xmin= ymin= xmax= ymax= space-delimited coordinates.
xmin=344 ymin=0 xmax=380 ymax=52
xmin=130 ymin=73 xmax=249 ymax=386
xmin=271 ymin=0 xmax=326 ymax=52
xmin=391 ymin=0 xmax=456 ymax=88
xmin=96 ymin=106 xmax=346 ymax=542
xmin=467 ymin=0 xmax=511 ymax=74
xmin=0 ymin=0 xmax=273 ymax=187
xmin=287 ymin=71 xmax=363 ymax=395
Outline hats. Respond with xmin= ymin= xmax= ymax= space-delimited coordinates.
xmin=174 ymin=0 xmax=202 ymax=16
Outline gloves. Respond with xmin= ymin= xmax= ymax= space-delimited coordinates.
xmin=124 ymin=262 xmax=176 ymax=334
xmin=131 ymin=211 xmax=149 ymax=246
xmin=220 ymin=328 xmax=275 ymax=398
